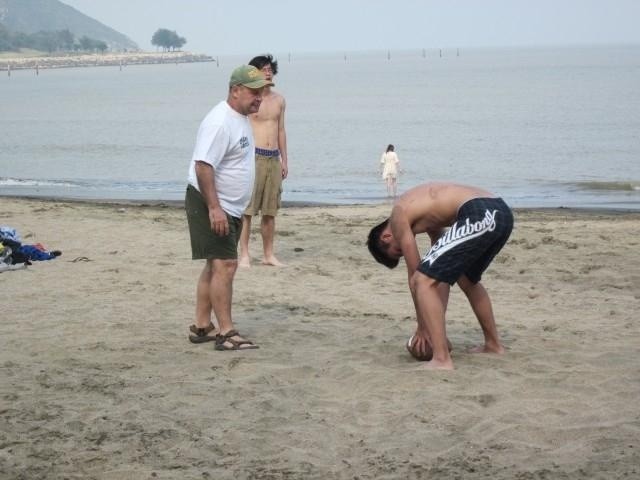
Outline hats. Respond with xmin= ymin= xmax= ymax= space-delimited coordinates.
xmin=229 ymin=64 xmax=276 ymax=90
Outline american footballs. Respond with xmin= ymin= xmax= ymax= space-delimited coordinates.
xmin=406 ymin=333 xmax=453 ymax=362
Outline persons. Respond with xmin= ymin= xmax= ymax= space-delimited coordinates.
xmin=237 ymin=54 xmax=289 ymax=268
xmin=378 ymin=143 xmax=405 ymax=192
xmin=185 ymin=64 xmax=274 ymax=351
xmin=364 ymin=181 xmax=513 ymax=371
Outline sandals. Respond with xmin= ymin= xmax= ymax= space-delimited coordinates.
xmin=188 ymin=321 xmax=260 ymax=351
